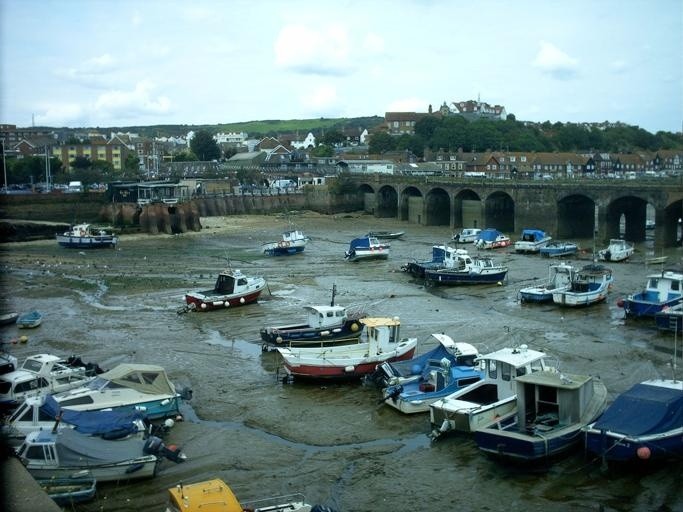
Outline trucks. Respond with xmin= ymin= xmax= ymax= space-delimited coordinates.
xmin=69 ymin=181 xmax=83 ymax=192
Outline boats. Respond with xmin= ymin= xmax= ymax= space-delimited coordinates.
xmin=577 ymin=322 xmax=683 ymax=468
xmin=165 ymin=479 xmax=334 ymax=512
xmin=552 ymin=229 xmax=614 ymax=307
xmin=540 ymin=238 xmax=579 ymax=258
xmin=644 ymin=256 xmax=669 ymax=265
xmin=377 ymin=358 xmax=485 ymax=416
xmin=258 ymin=283 xmax=372 ymax=348
xmin=514 ymin=229 xmax=552 ymax=254
xmin=400 ymin=227 xmax=512 ymax=286
xmin=473 ymin=368 xmax=608 ymax=475
xmin=365 ymin=230 xmax=406 ymax=240
xmin=345 ymin=236 xmax=391 ymax=262
xmin=623 ymin=246 xmax=683 ymax=316
xmin=360 ymin=332 xmax=484 ymax=393
xmin=0 ymin=312 xmax=18 ymax=327
xmin=654 ymin=302 xmax=683 ymax=335
xmin=261 ymin=229 xmax=310 ymax=257
xmin=597 ymin=238 xmax=635 ymax=263
xmin=34 ymin=476 xmax=97 ymax=504
xmin=176 ymin=244 xmax=268 ymax=314
xmin=16 ymin=309 xmax=43 ymax=328
xmin=56 ymin=221 xmax=119 ymax=249
xmin=517 ymin=262 xmax=580 ymax=302
xmin=276 ymin=316 xmax=419 ymax=382
xmin=1 ymin=348 xmax=194 ymax=483
xmin=428 ymin=326 xmax=560 ymax=445
